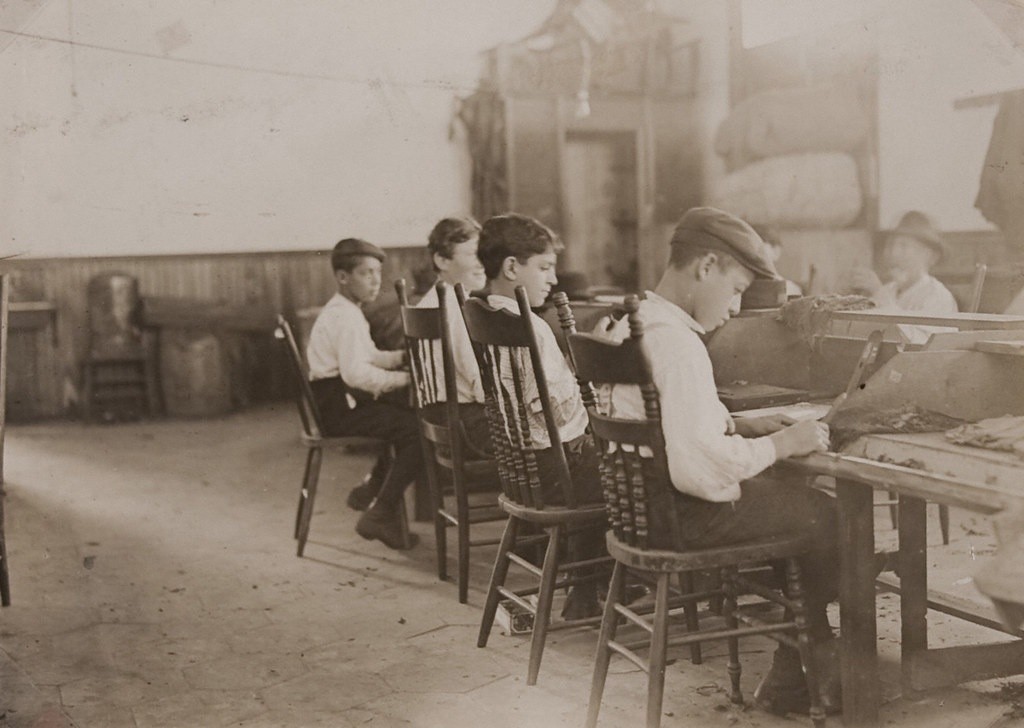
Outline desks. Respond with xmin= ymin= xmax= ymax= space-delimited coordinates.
xmin=714 ymin=381 xmax=1024 ymax=728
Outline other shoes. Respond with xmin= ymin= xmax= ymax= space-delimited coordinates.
xmin=563 ymin=583 xmax=647 ymax=618
xmin=344 ymin=480 xmax=384 ymax=510
xmin=755 ymin=647 xmax=847 ymax=715
xmin=357 ymin=508 xmax=419 ymax=551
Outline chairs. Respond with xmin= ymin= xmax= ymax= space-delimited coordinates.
xmin=554 ymin=289 xmax=827 ymax=728
xmin=79 ymin=271 xmax=158 ymax=423
xmin=454 ymin=281 xmax=702 ymax=685
xmin=277 ymin=314 xmax=414 ymax=556
xmin=394 ymin=275 xmax=573 ymax=603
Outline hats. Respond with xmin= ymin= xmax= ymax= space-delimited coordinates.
xmin=670 ymin=206 xmax=776 ymax=279
xmin=336 ymin=238 xmax=387 ymax=264
xmin=890 ymin=209 xmax=944 ymax=257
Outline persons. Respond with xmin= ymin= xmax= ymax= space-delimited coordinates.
xmin=613 ymin=208 xmax=841 ymax=714
xmin=849 ymin=212 xmax=959 ymax=343
xmin=307 ymin=237 xmax=421 ymax=548
xmin=748 ymin=220 xmax=803 ymax=296
xmin=416 ymin=218 xmax=496 ymax=459
xmin=477 ymin=212 xmax=607 ymax=503
